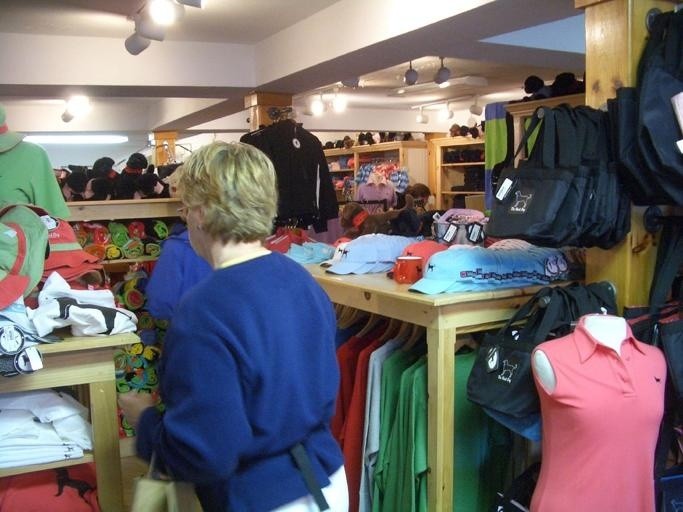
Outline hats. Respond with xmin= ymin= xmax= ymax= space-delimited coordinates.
xmin=281 ymin=241 xmax=337 ymax=266
xmin=407 ymin=238 xmax=586 ymax=296
xmin=319 ymin=232 xmax=450 ymax=277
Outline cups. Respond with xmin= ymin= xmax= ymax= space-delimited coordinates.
xmin=395 ymin=256 xmax=423 ymax=283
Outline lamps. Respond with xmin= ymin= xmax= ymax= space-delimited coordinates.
xmin=403 ymin=58 xmax=419 ymax=87
xmin=131 ymin=0 xmax=204 ymax=44
xmin=432 ymin=54 xmax=453 ymax=86
xmin=412 ymin=94 xmax=483 ymax=126
xmin=297 ymin=85 xmax=346 ymax=116
xmin=340 ymin=77 xmax=360 ymax=88
xmin=123 ymin=29 xmax=154 ymax=56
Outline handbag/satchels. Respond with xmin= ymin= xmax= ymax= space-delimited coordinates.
xmin=124 ymin=415 xmax=208 ymax=511
xmin=483 ymin=101 xmax=634 ymax=252
xmin=466 ymin=282 xmax=620 ymax=443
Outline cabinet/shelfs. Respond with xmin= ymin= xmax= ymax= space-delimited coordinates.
xmin=64 ymin=195 xmax=188 ymax=441
xmin=354 ymin=139 xmax=430 ymax=198
xmin=500 ymin=90 xmax=587 ymax=170
xmin=430 ymin=132 xmax=485 ymax=214
xmin=0 ymin=330 xmax=141 ymax=511
xmin=322 ymin=146 xmax=356 ymax=206
xmin=292 ymin=256 xmax=593 ymax=512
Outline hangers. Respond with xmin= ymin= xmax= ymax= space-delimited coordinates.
xmin=328 ymin=298 xmax=480 ymax=361
xmin=266 ymin=106 xmax=297 ymax=127
xmin=272 ymin=212 xmax=323 ymax=234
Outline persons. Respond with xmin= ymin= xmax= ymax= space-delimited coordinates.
xmin=530 ymin=313 xmax=668 ymax=512
xmin=145 ymin=222 xmax=216 ymax=319
xmin=0 ymin=104 xmax=70 ymax=220
xmin=408 ymin=183 xmax=431 ymax=212
xmin=117 ymin=140 xmax=350 ymax=512
